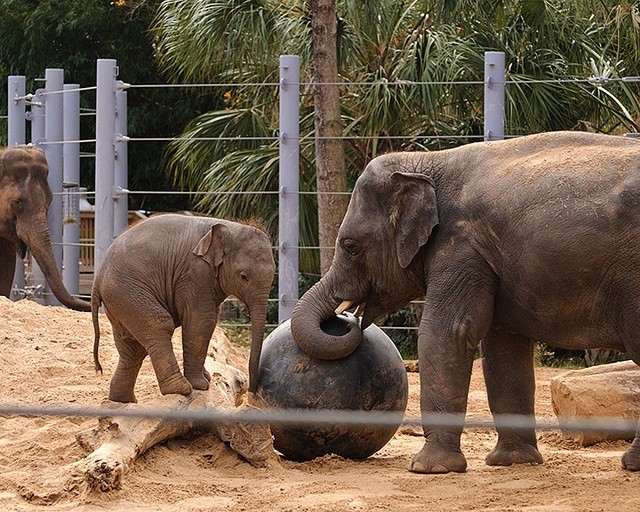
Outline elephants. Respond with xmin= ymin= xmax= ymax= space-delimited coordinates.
xmin=0 ymin=146 xmax=90 ymax=312
xmin=91 ymin=213 xmax=277 ymax=404
xmin=291 ymin=129 xmax=640 ymax=474
